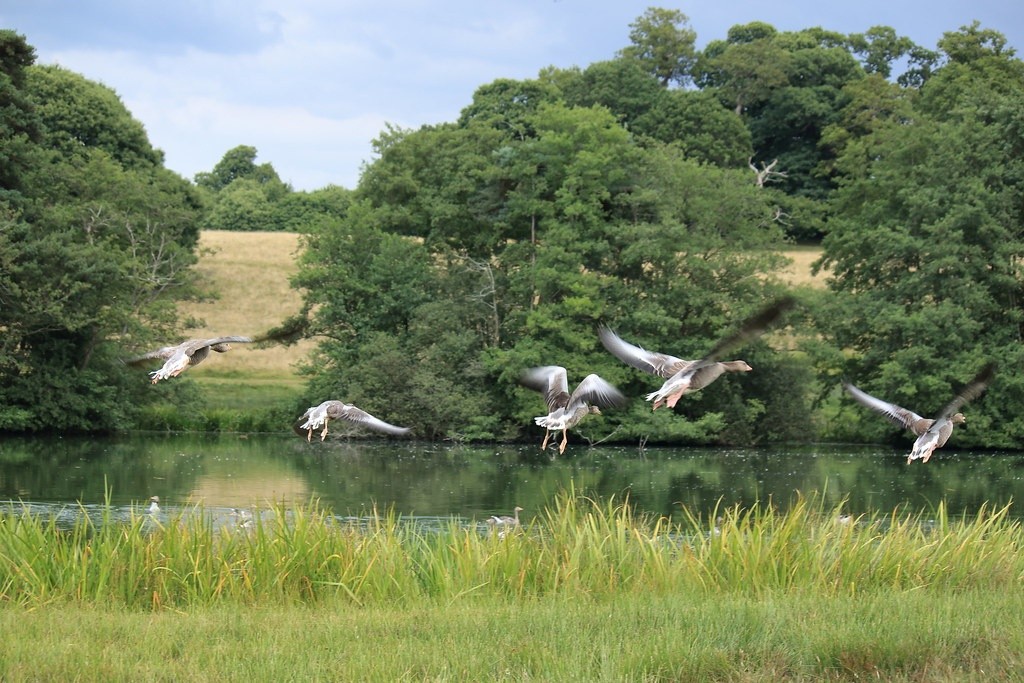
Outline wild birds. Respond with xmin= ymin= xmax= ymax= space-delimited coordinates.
xmin=506 ymin=366 xmax=633 ymax=455
xmin=126 ymin=327 xmax=303 ymax=386
xmin=836 ymin=359 xmax=1001 ymax=466
xmin=588 ymin=296 xmax=800 ymax=413
xmin=229 ymin=508 xmax=253 ymax=530
xmin=297 ymin=399 xmax=421 ymax=443
xmin=149 ymin=492 xmax=160 ymax=511
xmin=477 ymin=489 xmax=856 ymax=553
